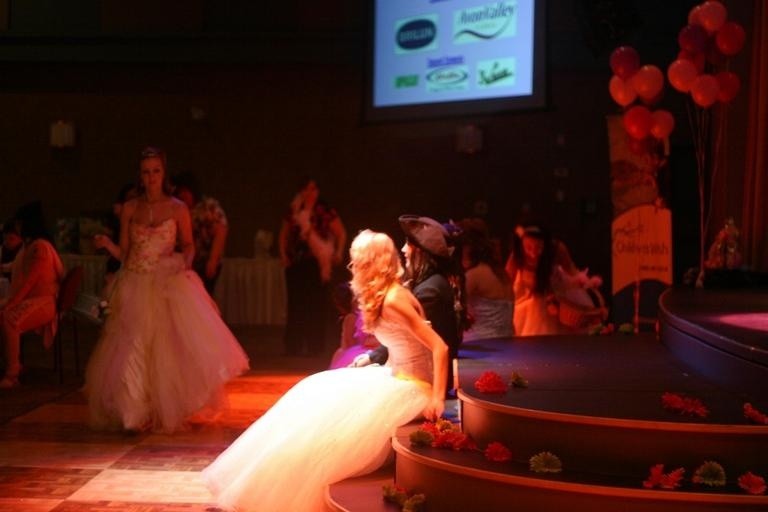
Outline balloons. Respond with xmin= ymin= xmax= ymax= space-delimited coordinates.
xmin=609 ymin=0 xmax=747 ymax=143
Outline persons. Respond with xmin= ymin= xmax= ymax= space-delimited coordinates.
xmin=1 ymin=200 xmax=62 ymax=392
xmin=432 ymin=219 xmax=515 ymax=337
xmin=346 ymin=211 xmax=460 ymax=399
xmin=103 ymin=174 xmax=230 ymax=296
xmin=503 ymin=217 xmax=579 ymax=336
xmin=278 ymin=176 xmax=347 ymax=360
xmin=93 ymin=144 xmax=197 ymax=434
xmin=199 ymin=229 xmax=450 ymax=510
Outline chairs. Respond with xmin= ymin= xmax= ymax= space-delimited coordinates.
xmin=21 ymin=265 xmax=84 ymax=384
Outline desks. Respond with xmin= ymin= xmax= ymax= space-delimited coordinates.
xmin=223 ymin=257 xmax=287 ymax=326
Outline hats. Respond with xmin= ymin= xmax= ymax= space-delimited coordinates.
xmin=397 ymin=213 xmax=457 ymax=260
